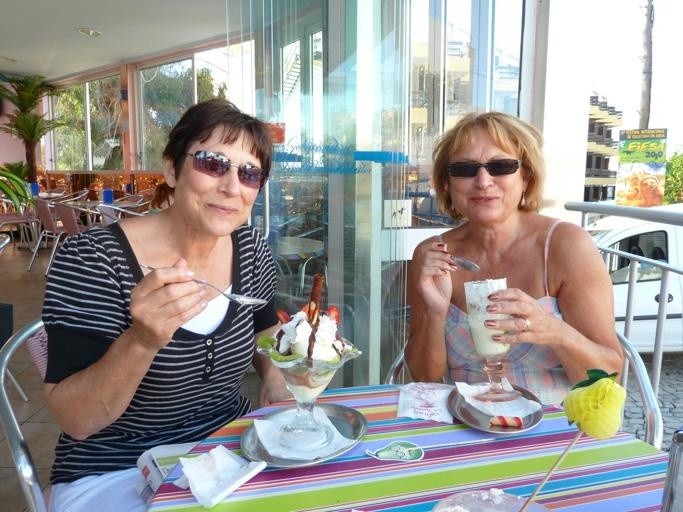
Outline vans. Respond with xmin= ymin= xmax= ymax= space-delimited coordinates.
xmin=582 ymin=202 xmax=683 ymax=355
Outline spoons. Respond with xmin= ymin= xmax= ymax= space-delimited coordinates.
xmin=138 ymin=259 xmax=270 ymax=311
xmin=452 ymin=255 xmax=481 ymax=273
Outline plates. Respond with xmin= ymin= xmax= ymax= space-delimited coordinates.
xmin=446 ymin=380 xmax=544 ymax=437
xmin=429 ymin=489 xmax=552 ymax=512
xmin=241 ymin=399 xmax=364 ymax=473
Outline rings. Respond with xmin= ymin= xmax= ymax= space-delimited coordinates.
xmin=518 ymin=315 xmax=530 ymax=333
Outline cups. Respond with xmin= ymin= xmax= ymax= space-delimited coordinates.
xmin=102 ymin=188 xmax=112 ymax=203
xmin=28 ymin=182 xmax=38 ymax=195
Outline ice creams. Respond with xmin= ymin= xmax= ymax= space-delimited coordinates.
xmin=255 ymin=274 xmax=361 ymax=401
xmin=467 ymin=312 xmax=511 ymax=356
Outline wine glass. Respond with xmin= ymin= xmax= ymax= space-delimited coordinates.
xmin=253 ymin=334 xmax=360 ymax=450
xmin=461 ymin=279 xmax=523 ymax=403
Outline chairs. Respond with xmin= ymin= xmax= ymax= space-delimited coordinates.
xmin=248 ymin=183 xmax=326 ymax=312
xmin=0 ymin=186 xmax=161 ymax=274
xmin=0 ymin=314 xmax=56 ymax=512
xmin=382 ymin=329 xmax=664 ymax=451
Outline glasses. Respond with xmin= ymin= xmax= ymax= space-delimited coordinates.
xmin=187 ymin=151 xmax=268 ymax=189
xmin=443 ymin=159 xmax=522 ymax=176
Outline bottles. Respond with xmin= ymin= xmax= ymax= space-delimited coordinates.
xmin=660 ymin=428 xmax=683 ymax=511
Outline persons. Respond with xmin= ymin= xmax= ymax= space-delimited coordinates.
xmin=41 ymin=98 xmax=294 ymax=511
xmin=402 ymin=111 xmax=624 ymax=408
